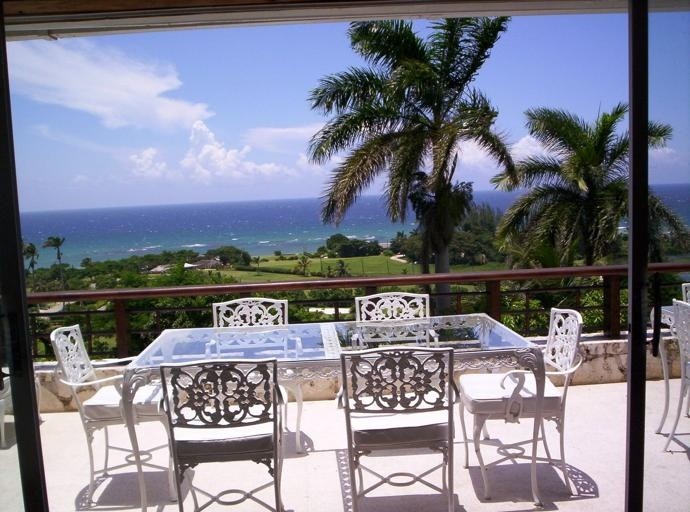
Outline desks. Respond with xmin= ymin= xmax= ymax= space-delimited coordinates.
xmin=119 ymin=308 xmax=547 ymax=511
xmin=647 ymin=301 xmax=690 ymax=436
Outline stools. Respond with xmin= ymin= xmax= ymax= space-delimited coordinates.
xmin=0 ymin=373 xmax=43 ymax=450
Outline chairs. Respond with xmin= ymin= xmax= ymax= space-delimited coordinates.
xmin=351 ymin=290 xmax=433 ymax=401
xmin=153 ymin=358 xmax=292 ymax=512
xmin=662 ymin=298 xmax=690 ymax=457
xmin=456 ymin=305 xmax=586 ymax=503
xmin=47 ymin=322 xmax=180 ymax=511
xmin=337 ymin=345 xmax=459 ymax=511
xmin=206 ymin=295 xmax=305 ymax=456
xmin=679 ymin=279 xmax=690 ymax=418
